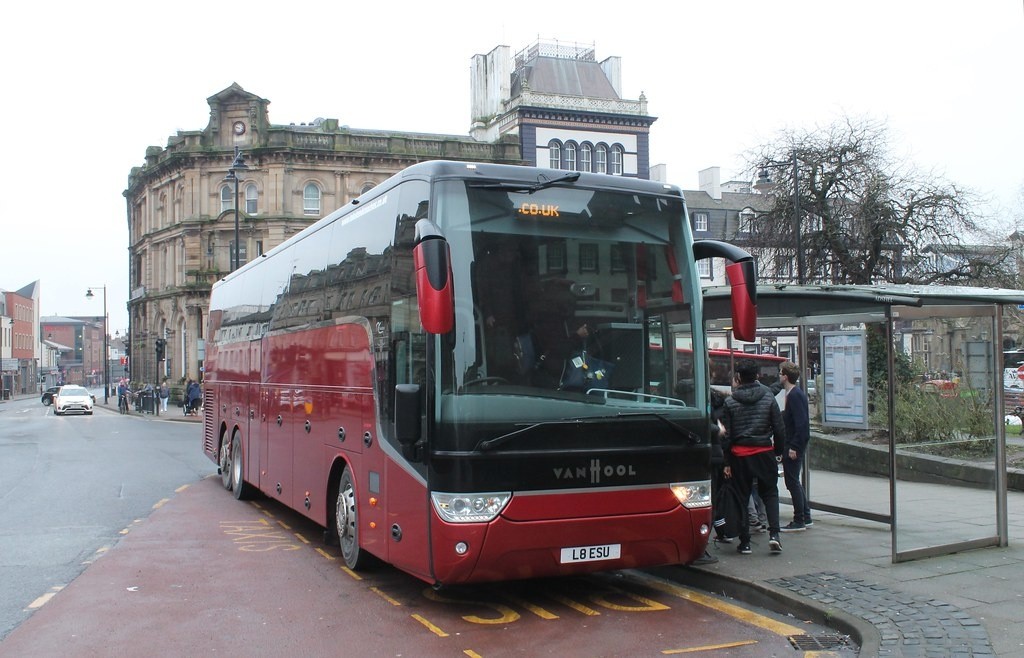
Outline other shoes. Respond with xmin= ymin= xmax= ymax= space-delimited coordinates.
xmin=694 ymin=551 xmax=719 ymax=564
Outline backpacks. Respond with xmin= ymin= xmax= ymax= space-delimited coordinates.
xmin=513 ymin=332 xmax=536 ymax=375
xmin=713 ymin=472 xmax=749 ymax=538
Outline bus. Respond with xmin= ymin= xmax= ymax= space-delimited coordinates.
xmin=1003 ymin=351 xmax=1024 ymax=394
xmin=201 ymin=160 xmax=758 ymax=595
xmin=648 ymin=343 xmax=789 ymax=396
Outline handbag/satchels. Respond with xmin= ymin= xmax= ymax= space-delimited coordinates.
xmin=559 ymin=328 xmax=615 ymax=393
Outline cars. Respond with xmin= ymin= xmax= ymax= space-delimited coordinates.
xmin=41 ymin=386 xmax=60 ymax=407
xmin=53 ymin=386 xmax=94 ymax=415
xmin=796 ymin=379 xmax=817 ymax=402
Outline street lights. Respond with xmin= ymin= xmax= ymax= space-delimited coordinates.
xmin=40 ymin=330 xmax=53 ymax=395
xmin=85 ymin=285 xmax=109 ymax=404
xmin=221 ymin=144 xmax=251 ymax=273
xmin=0 ymin=316 xmax=16 ymax=398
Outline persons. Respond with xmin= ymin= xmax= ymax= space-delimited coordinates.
xmin=56 ymin=377 xmax=64 ymax=386
xmin=119 ymin=376 xmax=130 ymax=386
xmin=780 ymin=361 xmax=813 ymax=532
xmin=185 ymin=377 xmax=201 ymax=415
xmin=940 ymin=370 xmax=949 ymax=380
xmin=481 ymin=241 xmax=533 ymax=385
xmin=117 ymin=382 xmax=156 ymax=413
xmin=678 ymin=355 xmax=726 ymax=564
xmin=732 ymin=372 xmax=784 ymax=533
xmin=922 ymin=371 xmax=929 ymax=381
xmin=160 ymin=381 xmax=171 ymax=412
xmin=1009 ymin=406 xmax=1024 ymax=426
xmin=931 ymin=370 xmax=939 ymax=380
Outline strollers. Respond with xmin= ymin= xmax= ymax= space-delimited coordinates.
xmin=183 ymin=395 xmax=196 ymax=416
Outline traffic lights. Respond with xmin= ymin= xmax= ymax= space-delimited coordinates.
xmin=155 ymin=338 xmax=166 ymax=359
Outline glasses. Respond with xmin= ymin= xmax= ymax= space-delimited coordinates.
xmin=778 ymin=372 xmax=785 ymax=376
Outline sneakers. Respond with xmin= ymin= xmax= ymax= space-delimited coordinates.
xmin=749 ymin=522 xmax=767 ymax=533
xmin=736 ymin=544 xmax=753 ymax=554
xmin=712 ymin=534 xmax=734 ymax=541
xmin=780 ymin=518 xmax=813 ymax=532
xmin=769 ymin=533 xmax=783 ymax=551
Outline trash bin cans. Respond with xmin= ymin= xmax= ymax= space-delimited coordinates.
xmin=23 ymin=388 xmax=26 ymax=394
xmin=111 ymin=386 xmax=117 ymax=396
xmin=140 ymin=392 xmax=153 ymax=412
xmin=4 ymin=389 xmax=10 ymax=400
